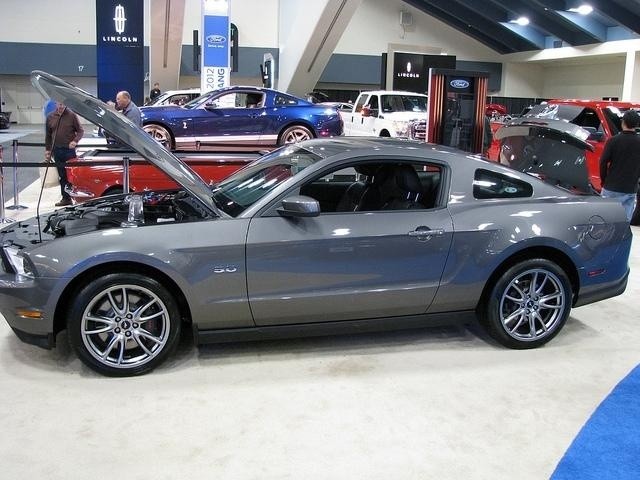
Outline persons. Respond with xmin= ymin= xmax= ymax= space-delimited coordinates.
xmin=116 ymin=89 xmax=144 ymax=130
xmin=43 ymin=101 xmax=85 ymax=206
xmin=150 ymin=82 xmax=161 ymax=101
xmin=598 ymin=109 xmax=640 ymax=223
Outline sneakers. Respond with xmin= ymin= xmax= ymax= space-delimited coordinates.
xmin=55 ymin=197 xmax=72 ymax=206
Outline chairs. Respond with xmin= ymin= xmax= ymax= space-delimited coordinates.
xmin=334 ymin=161 xmax=426 ymax=210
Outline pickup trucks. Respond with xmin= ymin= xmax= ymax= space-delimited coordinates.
xmin=336 ymin=89 xmax=427 ymax=142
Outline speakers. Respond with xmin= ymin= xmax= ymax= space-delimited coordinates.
xmin=423 ymin=55 xmax=457 ymax=69
xmin=400 ymin=11 xmax=412 ymax=26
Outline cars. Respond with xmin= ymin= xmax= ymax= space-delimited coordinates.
xmin=64 ymin=150 xmax=291 ymax=204
xmin=0 ymin=71 xmax=632 ymax=377
xmin=97 ymin=85 xmax=345 ymax=146
xmin=91 ymin=88 xmax=201 ymax=138
xmin=483 ymin=99 xmax=640 ymax=225
